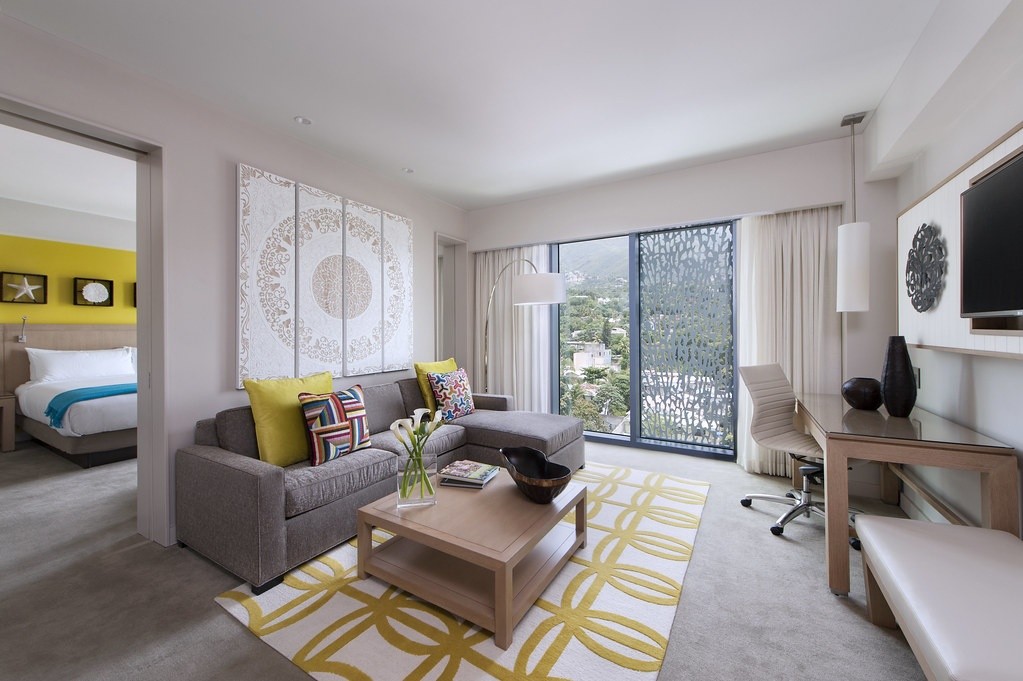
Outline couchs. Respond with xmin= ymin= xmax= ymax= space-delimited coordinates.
xmin=171 ymin=378 xmax=585 ymax=596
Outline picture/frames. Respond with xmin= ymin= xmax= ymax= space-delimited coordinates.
xmin=0 ymin=271 xmax=48 ymax=304
xmin=73 ymin=277 xmax=114 ymax=307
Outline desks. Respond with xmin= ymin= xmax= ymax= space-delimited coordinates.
xmin=794 ymin=392 xmax=1019 ymax=596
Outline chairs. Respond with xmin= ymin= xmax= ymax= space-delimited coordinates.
xmin=737 ymin=364 xmax=865 ymax=551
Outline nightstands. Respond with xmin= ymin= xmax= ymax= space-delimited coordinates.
xmin=0 ymin=391 xmax=16 ymax=452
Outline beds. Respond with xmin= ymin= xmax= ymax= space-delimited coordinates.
xmin=0 ymin=323 xmax=138 ymax=465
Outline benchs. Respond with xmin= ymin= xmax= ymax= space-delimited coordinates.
xmin=854 ymin=512 xmax=1023 ymax=681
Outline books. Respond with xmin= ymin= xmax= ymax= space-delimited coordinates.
xmin=440 ymin=476 xmax=495 ymax=489
xmin=438 ymin=460 xmax=501 ymax=484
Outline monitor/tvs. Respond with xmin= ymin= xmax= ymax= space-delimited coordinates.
xmin=959 ymin=153 xmax=1023 ymax=318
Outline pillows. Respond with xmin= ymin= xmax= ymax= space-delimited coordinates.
xmin=24 ymin=347 xmax=138 ymax=381
xmin=428 ymin=368 xmax=475 ymax=423
xmin=300 ymin=385 xmax=371 ymax=465
xmin=413 ymin=357 xmax=456 ymax=418
xmin=245 ymin=372 xmax=333 ymax=465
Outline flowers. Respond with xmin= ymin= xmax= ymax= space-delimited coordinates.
xmin=390 ymin=407 xmax=442 ymax=497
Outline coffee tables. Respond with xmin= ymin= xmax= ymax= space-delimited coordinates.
xmin=357 ymin=461 xmax=589 ymax=652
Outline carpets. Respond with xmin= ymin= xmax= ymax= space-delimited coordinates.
xmin=215 ymin=459 xmax=709 ymax=681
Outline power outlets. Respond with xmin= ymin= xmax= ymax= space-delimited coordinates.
xmin=18 ymin=336 xmax=26 ymax=342
xmin=912 ymin=366 xmax=920 ymax=388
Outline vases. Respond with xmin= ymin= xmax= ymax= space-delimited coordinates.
xmin=882 ymin=336 xmax=917 ymax=419
xmin=396 ymin=455 xmax=437 ymax=508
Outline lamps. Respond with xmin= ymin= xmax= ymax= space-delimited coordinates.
xmin=833 ymin=112 xmax=871 ymax=314
xmin=483 ymin=257 xmax=568 ymax=392
